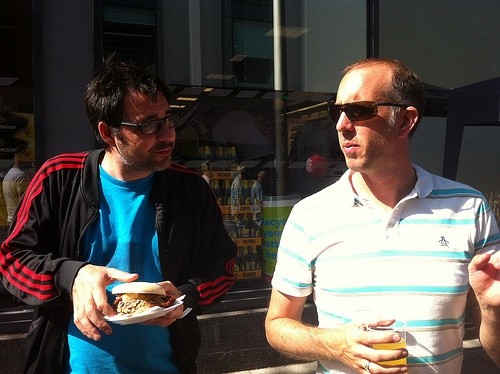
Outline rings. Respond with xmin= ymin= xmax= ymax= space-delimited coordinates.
xmin=364 ymin=361 xmax=370 ymax=371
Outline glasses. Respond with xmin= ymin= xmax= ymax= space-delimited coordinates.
xmin=328 ymin=101 xmax=411 ymax=124
xmin=119 ymin=111 xmax=179 ymax=135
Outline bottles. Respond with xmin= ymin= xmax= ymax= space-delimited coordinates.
xmin=192 ymin=143 xmax=262 ymax=272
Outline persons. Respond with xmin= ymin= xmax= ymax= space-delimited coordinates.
xmin=200 ymin=160 xmax=270 ymax=226
xmin=0 ymin=58 xmax=237 ymax=374
xmin=265 ymin=58 xmax=500 ymax=374
xmin=2 ymin=146 xmax=31 ymax=231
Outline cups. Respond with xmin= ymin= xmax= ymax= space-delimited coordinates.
xmin=366 ymin=321 xmax=407 ymax=374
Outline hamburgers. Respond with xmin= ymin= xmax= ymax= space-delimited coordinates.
xmin=112 ymin=281 xmax=175 ymax=317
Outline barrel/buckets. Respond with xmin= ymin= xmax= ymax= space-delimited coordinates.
xmin=263 ymin=194 xmax=303 ymax=283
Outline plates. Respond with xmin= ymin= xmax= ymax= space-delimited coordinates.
xmin=107 ymin=299 xmax=183 ymax=327
